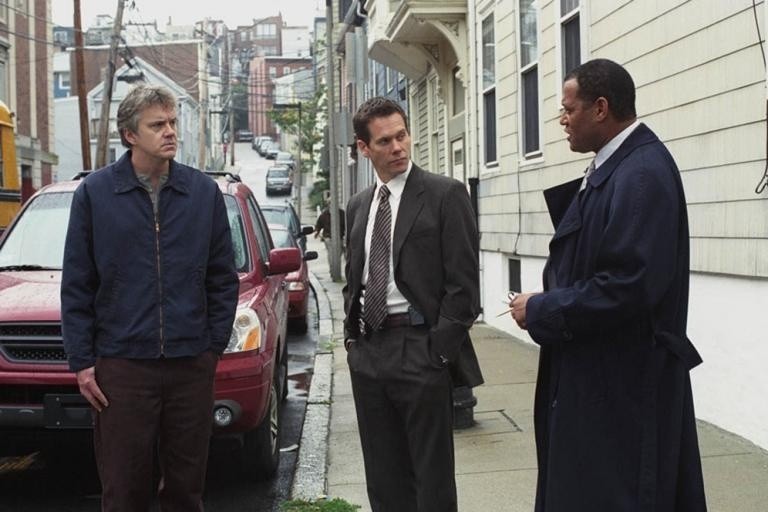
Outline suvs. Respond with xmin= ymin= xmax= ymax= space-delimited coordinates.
xmin=0 ymin=170 xmax=302 ymax=475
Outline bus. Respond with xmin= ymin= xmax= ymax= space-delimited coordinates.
xmin=0 ymin=100 xmax=25 ymax=237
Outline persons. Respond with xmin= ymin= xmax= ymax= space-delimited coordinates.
xmin=60 ymin=85 xmax=240 ymax=512
xmin=340 ymin=97 xmax=485 ymax=512
xmin=509 ymin=59 xmax=708 ymax=512
xmin=314 ymin=197 xmax=345 ymax=282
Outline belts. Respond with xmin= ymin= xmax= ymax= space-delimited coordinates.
xmin=352 ymin=307 xmax=434 ymax=335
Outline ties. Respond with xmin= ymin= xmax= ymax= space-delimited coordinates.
xmin=360 ymin=183 xmax=399 ymax=332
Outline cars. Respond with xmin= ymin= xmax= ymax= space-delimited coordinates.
xmin=260 ymin=200 xmax=316 ymax=331
xmin=233 ymin=126 xmax=299 ymax=195
